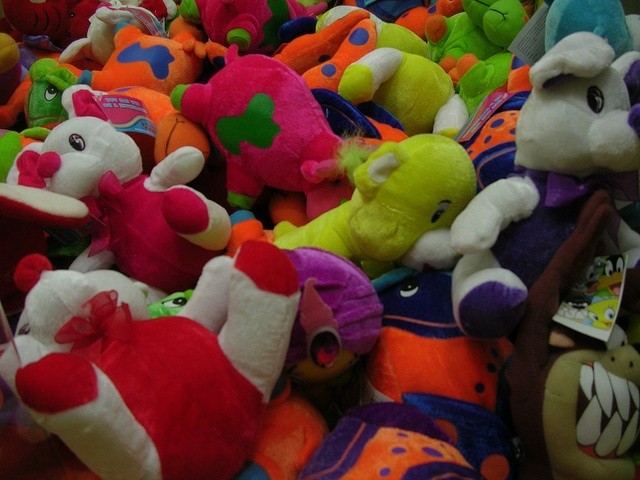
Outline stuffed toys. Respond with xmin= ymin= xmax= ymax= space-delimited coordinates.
xmin=0 ymin=0 xmax=640 ymax=480
xmin=0 ymin=2 xmax=638 ymax=480
